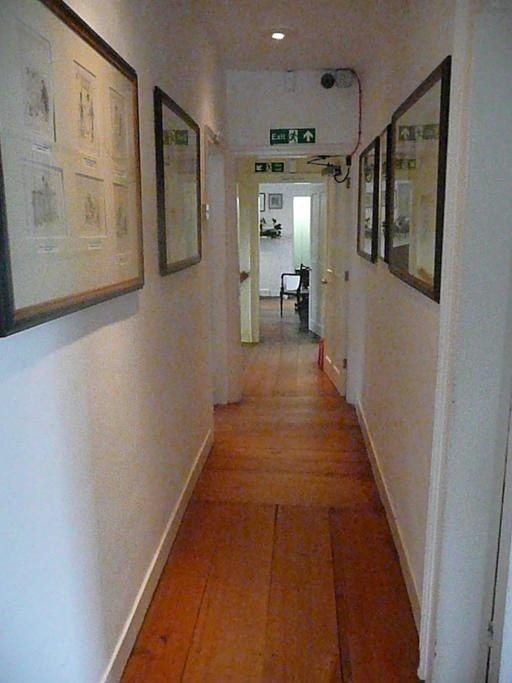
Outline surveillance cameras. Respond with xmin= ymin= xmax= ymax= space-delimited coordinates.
xmin=319 ymin=72 xmax=335 ymax=90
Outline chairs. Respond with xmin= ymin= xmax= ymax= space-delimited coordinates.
xmin=279 ymin=262 xmax=309 ymax=321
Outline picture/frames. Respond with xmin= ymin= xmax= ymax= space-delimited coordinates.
xmin=356 ymin=54 xmax=455 ymax=305
xmin=257 ymin=192 xmax=283 ymax=213
